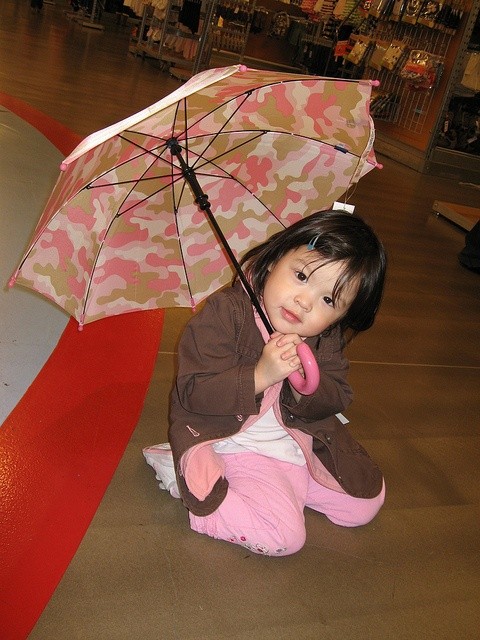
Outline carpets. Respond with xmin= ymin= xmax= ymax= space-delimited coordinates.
xmin=0 ymin=88 xmax=165 ymax=639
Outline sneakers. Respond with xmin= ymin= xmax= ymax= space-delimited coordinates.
xmin=141 ymin=442 xmax=182 ymax=499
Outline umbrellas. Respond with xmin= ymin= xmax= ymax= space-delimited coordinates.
xmin=4 ymin=64 xmax=383 ymax=397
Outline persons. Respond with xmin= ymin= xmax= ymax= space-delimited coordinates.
xmin=141 ymin=209 xmax=388 ymax=558
